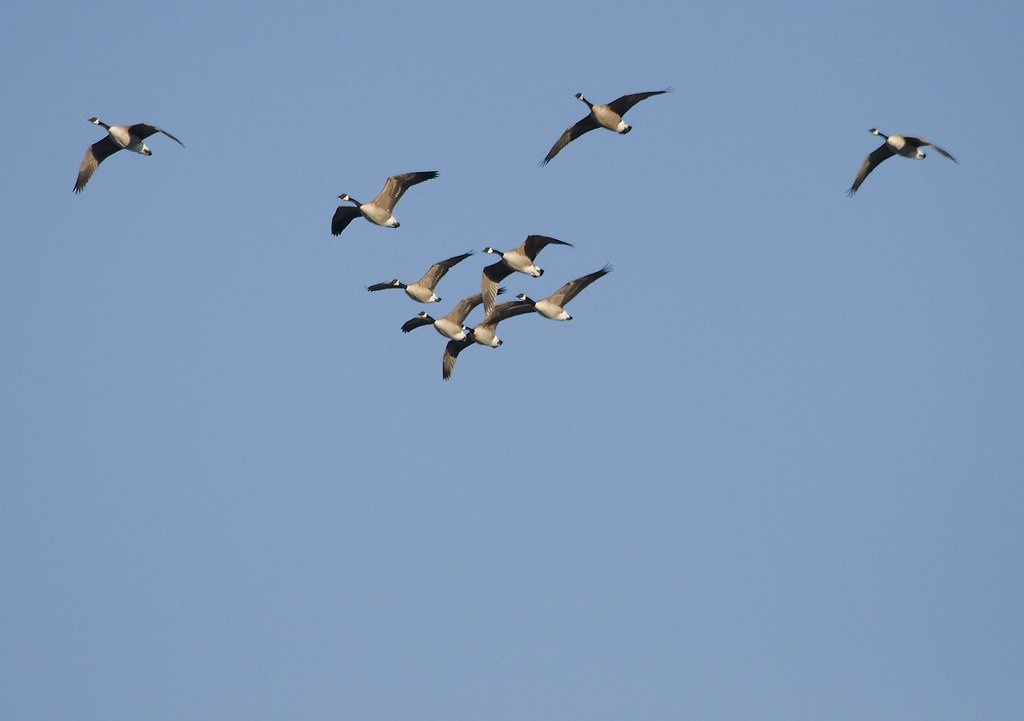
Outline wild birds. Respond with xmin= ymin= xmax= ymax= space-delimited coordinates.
xmin=539 ymin=84 xmax=672 ymax=170
xmin=365 ymin=232 xmax=616 ymax=384
xmin=330 ymin=170 xmax=440 ymax=238
xmin=72 ymin=116 xmax=187 ymax=196
xmin=846 ymin=126 xmax=962 ymax=200
